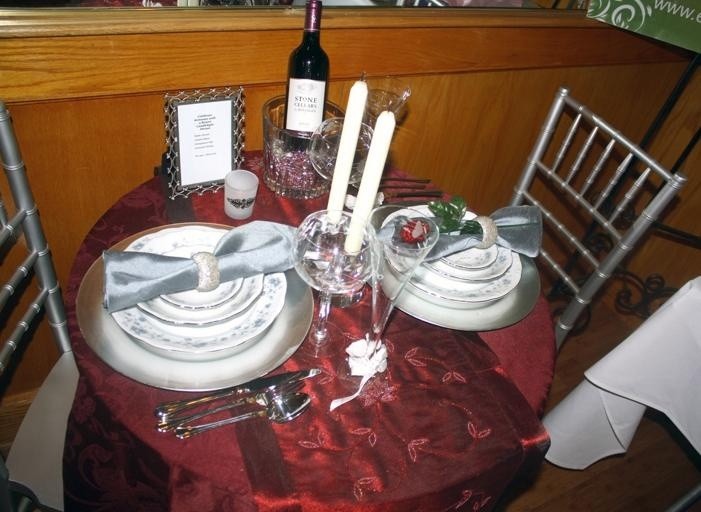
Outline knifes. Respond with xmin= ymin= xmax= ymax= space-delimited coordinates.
xmin=154 ymin=368 xmax=322 ymax=418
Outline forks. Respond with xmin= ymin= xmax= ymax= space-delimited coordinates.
xmin=155 ymin=375 xmax=307 ymax=433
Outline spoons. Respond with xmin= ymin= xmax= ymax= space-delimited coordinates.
xmin=173 ymin=392 xmax=312 ymax=440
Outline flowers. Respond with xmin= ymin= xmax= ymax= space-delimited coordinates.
xmin=395 ymin=187 xmax=546 ymax=259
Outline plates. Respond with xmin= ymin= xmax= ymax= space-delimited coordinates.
xmin=384 ymin=205 xmax=523 ymax=309
xmin=422 ymin=242 xmax=513 ymax=281
xmin=134 ymin=229 xmax=265 ymax=326
xmin=379 ymin=252 xmax=542 ymax=332
xmin=111 ymin=226 xmax=289 ymax=352
xmin=159 ymin=245 xmax=245 ymax=310
xmin=74 ymin=222 xmax=314 ymax=392
xmin=441 ymin=220 xmax=499 ymax=269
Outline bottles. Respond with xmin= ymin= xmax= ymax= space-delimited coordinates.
xmin=284 ymin=1 xmax=330 ymax=155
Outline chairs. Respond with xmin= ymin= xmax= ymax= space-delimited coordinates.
xmin=0 ymin=102 xmax=78 ymax=512
xmin=506 ymin=82 xmax=686 ymax=401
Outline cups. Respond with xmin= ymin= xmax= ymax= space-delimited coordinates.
xmin=262 ymin=94 xmax=347 ymax=198
xmin=337 ymin=204 xmax=440 ymax=397
xmin=224 ymin=169 xmax=259 ymax=220
xmin=361 ymin=73 xmax=412 ymax=146
xmin=292 ymin=208 xmax=382 ymax=359
xmin=309 ymin=117 xmax=375 ymax=184
